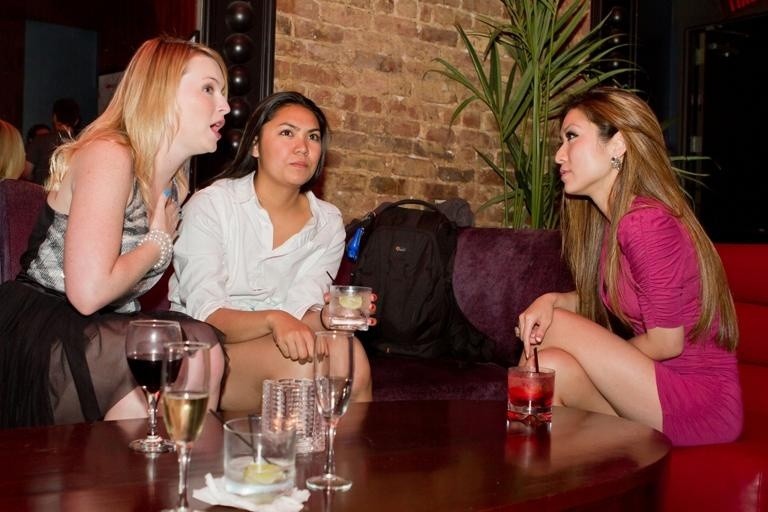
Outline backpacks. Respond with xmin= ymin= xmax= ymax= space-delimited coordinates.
xmin=350 ymin=199 xmax=456 ymax=360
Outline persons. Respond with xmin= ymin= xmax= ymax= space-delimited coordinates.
xmin=512 ymin=87 xmax=747 ymax=449
xmin=0 ymin=119 xmax=27 ymax=181
xmin=27 ymin=123 xmax=51 ymax=144
xmin=165 ymin=90 xmax=379 ymax=410
xmin=0 ymin=31 xmax=233 ymax=429
xmin=21 ymin=97 xmax=81 ymax=185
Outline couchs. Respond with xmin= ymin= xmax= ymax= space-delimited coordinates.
xmin=1 ymin=178 xmax=767 ymax=511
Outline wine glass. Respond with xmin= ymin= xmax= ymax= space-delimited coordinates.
xmin=306 ymin=377 xmax=353 ymax=491
xmin=125 ymin=320 xmax=211 ymax=512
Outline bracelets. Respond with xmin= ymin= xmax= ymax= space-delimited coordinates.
xmin=136 ymin=229 xmax=175 ymax=270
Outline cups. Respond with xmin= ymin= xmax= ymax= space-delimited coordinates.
xmin=507 ymin=367 xmax=555 ymax=435
xmin=329 ymin=285 xmax=371 ymax=331
xmin=223 ymin=418 xmax=296 ymax=495
xmin=260 ymin=378 xmax=315 ymax=458
